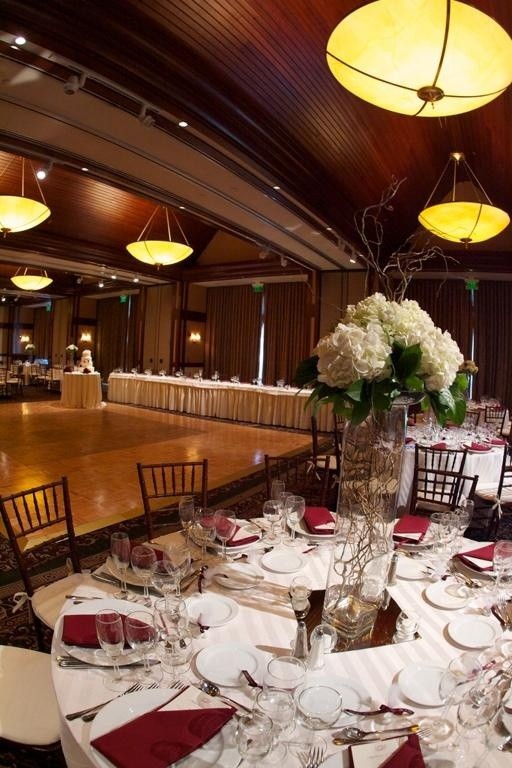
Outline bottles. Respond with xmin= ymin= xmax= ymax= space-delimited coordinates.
xmin=293 ymin=621 xmax=324 ymax=669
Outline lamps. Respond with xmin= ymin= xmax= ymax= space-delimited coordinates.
xmin=0 ymin=154 xmax=51 ymax=235
xmin=9 ymin=264 xmax=55 ymax=291
xmin=125 ymin=203 xmax=195 ymax=270
xmin=325 ymin=0 xmax=511 ymax=120
xmin=416 ymin=146 xmax=511 ymax=247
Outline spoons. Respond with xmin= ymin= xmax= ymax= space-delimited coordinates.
xmin=199 ymin=679 xmax=253 ymax=711
xmin=57 ymin=655 xmax=162 ymax=666
xmin=342 ymin=724 xmax=419 ymax=738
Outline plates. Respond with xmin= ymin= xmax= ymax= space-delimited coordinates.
xmin=180 ymin=593 xmax=240 ymax=626
xmin=106 ymin=553 xmax=172 ymax=588
xmin=426 ymin=583 xmax=477 ymax=609
xmin=288 ymin=511 xmax=511 ymax=577
xmin=54 ymin=599 xmax=160 ymax=666
xmin=447 ymin=614 xmax=502 ymax=649
xmin=191 ymin=518 xmax=262 ymax=548
xmin=89 ymin=689 xmax=227 ymax=768
xmin=196 ymin=643 xmax=264 ymax=687
xmin=335 ymin=546 xmax=363 ymax=562
xmin=396 ymin=552 xmax=432 ymax=580
xmin=262 ymin=550 xmax=307 ymax=574
xmin=407 ymin=437 xmax=508 ymax=453
xmin=294 ymin=679 xmax=370 ymax=728
xmin=397 ymin=663 xmax=451 ymax=707
xmin=214 ymin=562 xmax=267 ymax=588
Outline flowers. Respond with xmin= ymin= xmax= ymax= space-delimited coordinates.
xmin=290 ymin=290 xmax=471 ymax=618
xmin=64 ymin=344 xmax=79 ymax=352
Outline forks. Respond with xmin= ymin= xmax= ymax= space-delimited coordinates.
xmin=334 ymin=728 xmax=431 ymax=744
xmin=296 ymin=747 xmax=326 ymax=767
xmin=65 ymin=682 xmax=184 ymax=723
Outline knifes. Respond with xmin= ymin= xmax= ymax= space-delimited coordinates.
xmin=93 ymin=572 xmax=135 ymax=589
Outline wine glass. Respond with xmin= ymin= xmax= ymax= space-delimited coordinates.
xmin=405 ymin=416 xmax=497 ymax=446
xmin=96 ymin=597 xmax=192 ymax=686
xmin=263 ymin=480 xmax=305 ymax=545
xmin=114 ymin=368 xmax=291 ymax=390
xmin=112 ymin=533 xmax=191 ymax=613
xmin=430 ymin=499 xmax=511 ymax=603
xmin=179 ymin=497 xmax=237 ymax=576
xmin=309 ymin=625 xmax=337 ymax=655
xmin=239 ymin=656 xmax=343 ymax=768
xmin=419 ymin=632 xmax=512 ymax=768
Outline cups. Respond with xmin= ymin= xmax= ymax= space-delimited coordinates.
xmin=467 ymin=396 xmax=499 ymax=412
xmin=291 ymin=598 xmax=311 ymax=619
xmin=396 ymin=611 xmax=420 ymax=634
xmin=290 ymin=576 xmax=313 ymax=599
